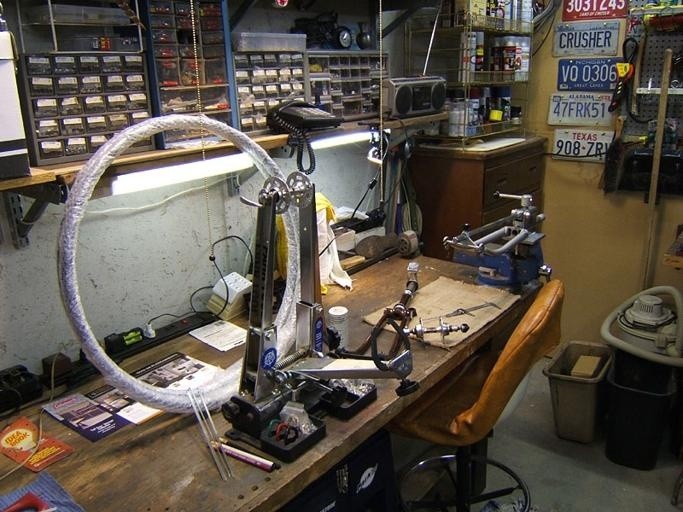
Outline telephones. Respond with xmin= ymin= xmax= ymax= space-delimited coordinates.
xmin=267 ymin=100 xmax=342 ymax=130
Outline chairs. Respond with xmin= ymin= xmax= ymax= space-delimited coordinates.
xmin=392 ymin=277 xmax=566 ymax=512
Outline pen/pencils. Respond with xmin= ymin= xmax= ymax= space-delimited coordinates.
xmin=208 ymin=437 xmax=281 ymax=473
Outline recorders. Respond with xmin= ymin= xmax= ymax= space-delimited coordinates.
xmin=382 ymin=75 xmax=446 ymax=120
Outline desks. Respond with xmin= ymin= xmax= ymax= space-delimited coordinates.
xmin=0 ymin=253 xmax=541 ymax=512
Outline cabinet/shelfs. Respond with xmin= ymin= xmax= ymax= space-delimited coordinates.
xmin=399 ymin=0 xmax=536 ymax=151
xmin=406 ymin=131 xmax=549 ymax=262
xmin=1 ymin=0 xmax=391 ymax=169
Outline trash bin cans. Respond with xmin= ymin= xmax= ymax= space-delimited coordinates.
xmin=606 ymin=350 xmax=676 ymax=471
xmin=542 ymin=341 xmax=613 ymax=443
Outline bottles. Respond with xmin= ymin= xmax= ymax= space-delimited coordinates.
xmin=490 ymin=46 xmax=522 ymax=81
xmin=91 ymin=37 xmax=111 ymax=51
xmin=328 ymin=305 xmax=350 ymax=350
xmin=356 ymin=22 xmax=374 ymax=50
xmin=444 ymin=87 xmax=522 ymax=136
xmin=486 ymin=0 xmax=505 ymax=29
